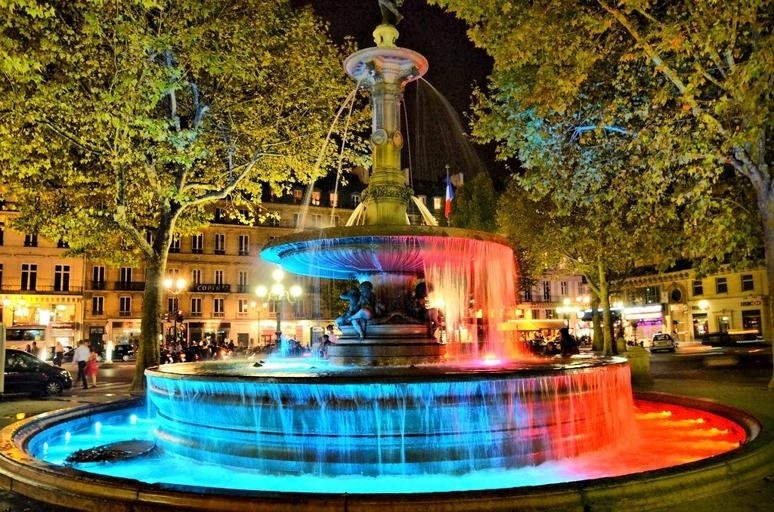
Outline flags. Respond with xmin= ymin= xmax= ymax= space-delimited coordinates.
xmin=444 ymin=173 xmax=455 ymax=218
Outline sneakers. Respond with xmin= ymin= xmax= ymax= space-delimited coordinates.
xmin=73 ymin=384 xmax=96 ymax=389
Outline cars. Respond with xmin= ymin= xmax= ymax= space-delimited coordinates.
xmin=52 ymin=343 xmax=234 ymax=370
xmin=649 ymin=332 xmax=676 ymax=351
xmin=0 ymin=349 xmax=72 ymax=400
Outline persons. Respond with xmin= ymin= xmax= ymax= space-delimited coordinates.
xmin=327 ymin=325 xmax=336 ymax=343
xmin=347 ymin=281 xmax=375 ymax=338
xmin=673 ymin=331 xmax=679 ymax=348
xmin=322 ymin=335 xmax=331 ymax=346
xmin=336 ymin=287 xmax=360 ymax=325
xmin=25 ymin=338 xmax=244 ymax=389
xmin=414 ymin=282 xmax=443 ymax=339
xmin=543 ymin=342 xmax=560 ymax=355
xmin=560 ymin=327 xmax=578 ymax=354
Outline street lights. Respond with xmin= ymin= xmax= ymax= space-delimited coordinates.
xmin=254 ymin=267 xmax=302 ymax=352
xmin=164 ymin=278 xmax=188 ymax=350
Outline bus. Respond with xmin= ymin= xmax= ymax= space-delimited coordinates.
xmin=5 ymin=325 xmax=49 ymax=361
